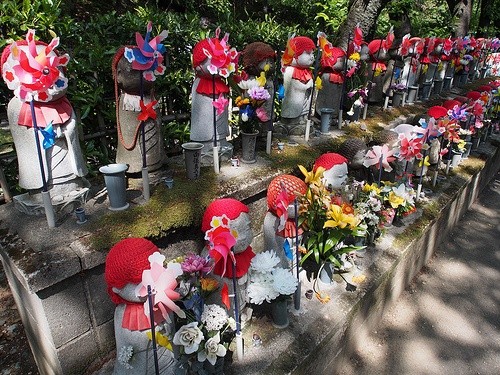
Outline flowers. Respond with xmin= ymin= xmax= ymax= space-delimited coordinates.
xmin=170 ymin=51 xmax=500 ymax=375
xmin=220 ymin=60 xmax=272 ymax=134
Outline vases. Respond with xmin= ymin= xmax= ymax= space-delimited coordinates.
xmin=181 ymin=141 xmax=205 ymax=180
xmin=461 ymin=71 xmax=469 ymax=85
xmin=320 ymin=259 xmax=335 ymax=286
xmin=318 ymin=106 xmax=335 ymax=134
xmin=480 ymin=68 xmax=489 ymax=80
xmin=461 ymin=141 xmax=472 ymax=160
xmin=368 ymin=226 xmax=374 ymax=247
xmin=481 ymin=128 xmax=488 ymax=142
xmin=407 ymin=85 xmax=418 ymax=104
xmin=269 ymin=301 xmax=289 ymax=330
xmin=351 ymin=105 xmax=360 ymax=122
xmin=239 ymin=129 xmax=260 ymax=163
xmin=453 ymin=73 xmax=462 ymax=88
xmin=472 ymin=136 xmax=480 ymax=151
xmin=443 ymin=76 xmax=453 ymax=91
xmin=99 ymin=162 xmax=131 ymax=212
xmin=384 ymin=212 xmax=396 ymax=227
xmin=452 ymin=154 xmax=462 ymax=168
xmin=468 ymin=71 xmax=476 ymax=82
xmin=488 ymin=124 xmax=494 ymax=135
xmin=433 ymin=79 xmax=443 ymax=95
xmin=392 ymin=90 xmax=404 ymax=106
xmin=421 ymin=82 xmax=432 ymax=98
